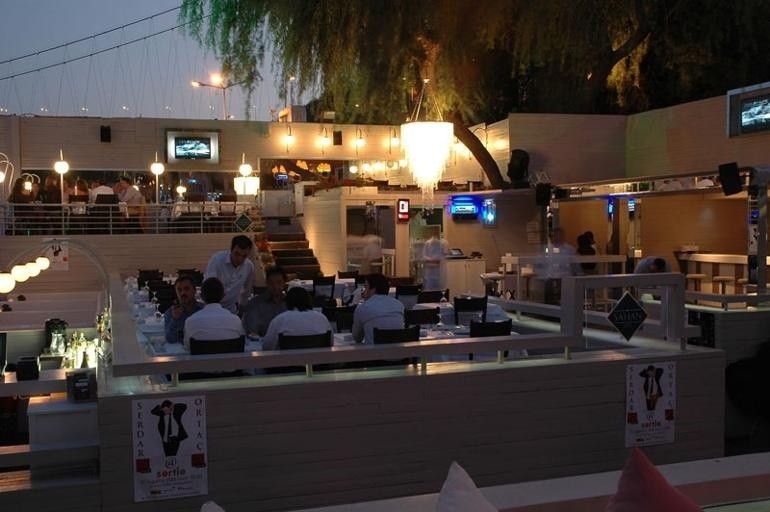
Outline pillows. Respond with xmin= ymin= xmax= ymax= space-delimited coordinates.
xmin=201 ymin=501 xmax=226 ymax=512
xmin=605 ymin=444 xmax=702 ymax=512
xmin=435 ymin=460 xmax=500 ymax=512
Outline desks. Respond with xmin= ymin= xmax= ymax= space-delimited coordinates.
xmin=498 ymin=253 xmax=628 ymax=306
xmin=680 ymin=251 xmax=770 ymax=311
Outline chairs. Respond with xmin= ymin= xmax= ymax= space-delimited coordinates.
xmin=309 ymin=173 xmax=495 ymax=193
xmin=20 ymin=180 xmax=255 ymax=234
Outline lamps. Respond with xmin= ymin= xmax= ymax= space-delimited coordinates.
xmin=55 ymin=149 xmax=73 ymax=235
xmin=238 ymin=152 xmax=255 ymax=219
xmin=151 ymin=149 xmax=167 ymax=234
xmin=389 ymin=126 xmax=401 ymax=158
xmin=318 ymin=127 xmax=333 ymax=156
xmin=396 ymin=63 xmax=458 ymax=200
xmin=354 ymin=126 xmax=366 ymax=159
xmin=284 ymin=123 xmax=297 ymax=154
xmin=1 ymin=152 xmax=43 ymax=194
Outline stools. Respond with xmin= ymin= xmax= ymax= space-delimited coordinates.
xmin=711 ymin=275 xmax=733 ymax=310
xmin=683 ymin=271 xmax=706 ymax=306
xmin=737 ymin=275 xmax=749 ymax=285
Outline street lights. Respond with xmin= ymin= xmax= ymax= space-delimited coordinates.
xmin=190 ymin=79 xmax=249 ymax=121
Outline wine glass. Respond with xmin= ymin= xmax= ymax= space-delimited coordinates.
xmin=151 ymin=291 xmax=159 ymax=307
xmin=440 ymin=289 xmax=448 ymax=307
xmin=154 ymin=303 xmax=162 ymax=322
xmin=143 ymin=280 xmax=150 ymax=294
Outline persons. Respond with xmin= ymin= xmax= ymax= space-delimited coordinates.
xmin=164 ymin=276 xmax=205 ymax=344
xmin=543 ymin=227 xmax=584 ymax=306
xmin=254 ymin=285 xmax=335 ymax=376
xmin=633 ymin=256 xmax=673 ymax=305
xmin=584 ymin=231 xmax=609 ymax=290
xmin=150 ymin=399 xmax=188 ymax=456
xmin=182 ymin=276 xmax=246 ymax=353
xmin=351 ymin=271 xmax=405 ymax=345
xmin=240 ymin=268 xmax=287 ymax=337
xmin=422 ymin=225 xmax=449 ymax=292
xmin=575 ymin=235 xmax=596 ymax=271
xmin=639 ymin=364 xmax=664 ymax=410
xmin=360 ymin=226 xmax=384 ymax=274
xmin=202 ymin=235 xmax=255 ymax=319
xmin=8 ymin=176 xmax=147 ymax=234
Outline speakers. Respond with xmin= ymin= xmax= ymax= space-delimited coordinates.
xmin=718 ymin=162 xmax=743 ymax=196
xmin=333 ymin=129 xmax=343 ymax=146
xmin=506 ymin=149 xmax=530 ymax=190
xmin=535 ymin=183 xmax=550 ymax=206
xmin=99 ymin=124 xmax=111 ymax=143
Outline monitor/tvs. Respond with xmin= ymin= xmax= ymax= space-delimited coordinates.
xmin=739 ymin=94 xmax=769 ymax=132
xmin=175 ymin=136 xmax=211 ymax=160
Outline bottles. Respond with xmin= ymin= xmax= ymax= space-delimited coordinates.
xmin=50 ymin=330 xmax=97 ymax=368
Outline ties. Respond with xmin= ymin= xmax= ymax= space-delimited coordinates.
xmin=168 ymin=414 xmax=172 ymax=436
xmin=649 ymin=378 xmax=654 ymax=395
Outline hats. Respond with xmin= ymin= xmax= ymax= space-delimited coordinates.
xmin=584 ymin=231 xmax=595 ymax=245
